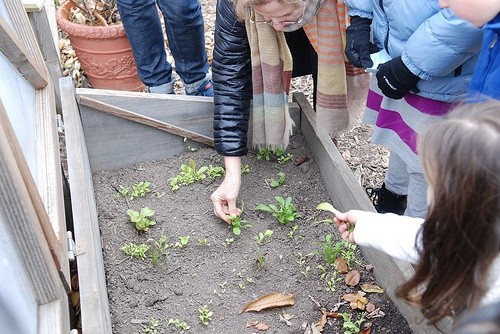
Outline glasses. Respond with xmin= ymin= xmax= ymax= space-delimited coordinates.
xmin=250 ymin=5 xmax=306 ymax=25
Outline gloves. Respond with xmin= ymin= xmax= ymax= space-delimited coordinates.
xmin=375 ymin=55 xmax=421 ymax=100
xmin=345 ymin=16 xmax=379 ymax=69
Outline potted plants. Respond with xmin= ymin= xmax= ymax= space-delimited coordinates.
xmin=55 ymin=0 xmax=150 ymax=92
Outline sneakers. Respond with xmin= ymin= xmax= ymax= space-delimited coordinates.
xmin=186 ymin=79 xmax=214 ymax=96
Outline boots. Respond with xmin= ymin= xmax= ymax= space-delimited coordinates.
xmin=366 ymin=182 xmax=408 ymax=214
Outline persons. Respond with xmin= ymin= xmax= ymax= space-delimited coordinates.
xmin=116 ymin=0 xmax=214 ymax=97
xmin=344 ymin=0 xmax=500 ymax=220
xmin=211 ymin=0 xmax=373 ymax=224
xmin=334 ymin=99 xmax=500 ymax=333
